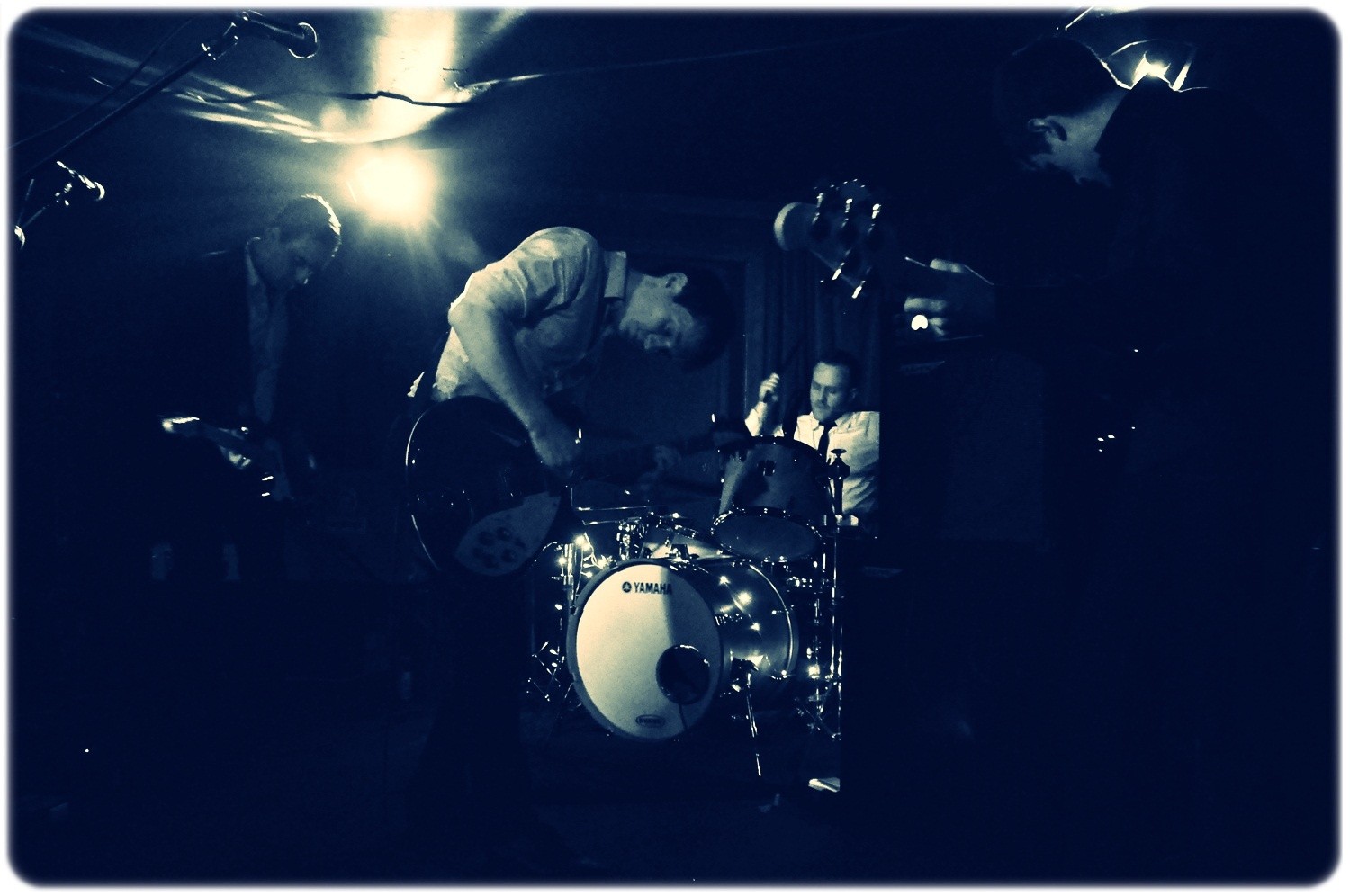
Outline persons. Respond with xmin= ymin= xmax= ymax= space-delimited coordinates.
xmin=155 ymin=195 xmax=350 ymax=742
xmin=742 ymin=345 xmax=888 ymax=542
xmin=381 ymin=224 xmax=740 ymax=756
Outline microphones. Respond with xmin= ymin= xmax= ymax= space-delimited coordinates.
xmin=56 ymin=161 xmax=105 ymax=201
xmin=237 ymin=10 xmax=320 ymax=58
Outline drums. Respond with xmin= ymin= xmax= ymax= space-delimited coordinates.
xmin=639 ymin=514 xmax=722 ymax=559
xmin=712 ymin=437 xmax=829 ymax=563
xmin=566 ymin=558 xmax=798 ymax=746
xmin=583 ymin=514 xmax=674 ymax=558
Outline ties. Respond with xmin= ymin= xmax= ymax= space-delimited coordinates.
xmin=818 ymin=421 xmax=837 ymax=461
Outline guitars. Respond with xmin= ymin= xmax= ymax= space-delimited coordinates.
xmin=409 ymin=395 xmax=723 ymax=585
xmin=772 ymin=180 xmax=930 ymax=303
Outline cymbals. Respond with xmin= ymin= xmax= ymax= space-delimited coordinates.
xmin=577 ymin=503 xmax=666 ymax=511
